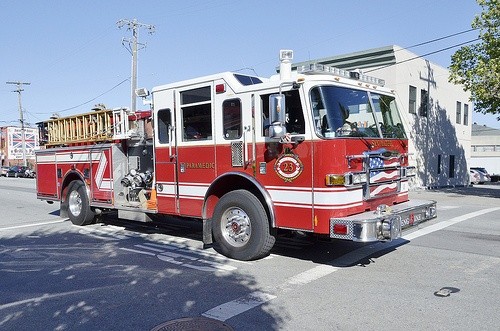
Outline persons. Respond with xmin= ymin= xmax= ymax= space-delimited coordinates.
xmin=325 ymin=102 xmax=352 ymax=133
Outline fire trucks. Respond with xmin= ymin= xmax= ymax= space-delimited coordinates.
xmin=33 ymin=48 xmax=437 ymax=261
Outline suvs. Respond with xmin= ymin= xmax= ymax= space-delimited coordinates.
xmin=6 ymin=165 xmax=30 ymax=177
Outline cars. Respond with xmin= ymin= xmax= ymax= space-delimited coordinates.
xmin=0 ymin=166 xmax=11 ymax=177
xmin=471 ymin=168 xmax=492 ymax=184
